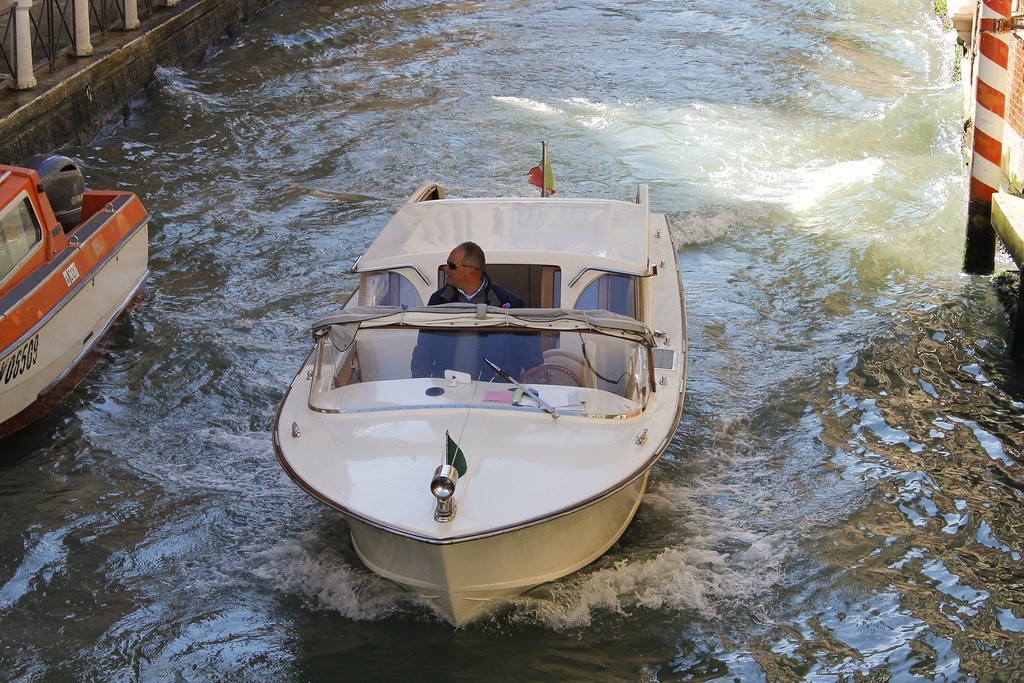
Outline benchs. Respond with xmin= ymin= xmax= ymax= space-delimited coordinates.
xmin=353 ymin=328 xmax=631 ymax=399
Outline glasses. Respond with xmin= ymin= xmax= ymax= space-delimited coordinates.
xmin=447 ymin=259 xmax=478 ymax=271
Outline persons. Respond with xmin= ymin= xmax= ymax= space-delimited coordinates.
xmin=411 ymin=241 xmax=545 ymax=384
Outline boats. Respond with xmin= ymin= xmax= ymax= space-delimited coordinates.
xmin=272 ymin=180 xmax=685 ymax=626
xmin=0 ymin=154 xmax=152 ymax=429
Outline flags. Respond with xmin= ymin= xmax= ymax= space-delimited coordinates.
xmin=523 ymin=159 xmax=557 ymax=195
